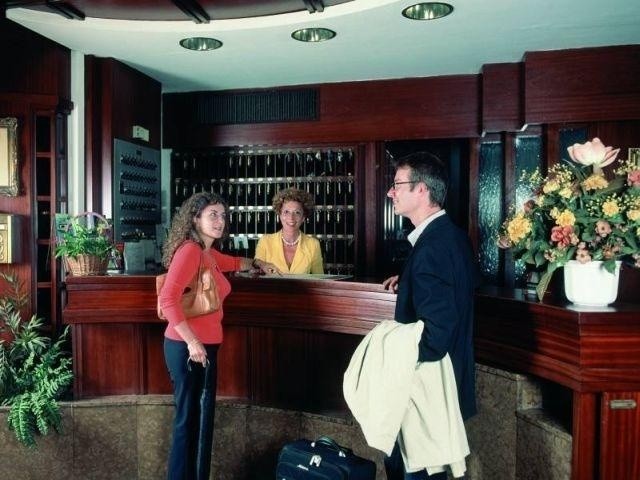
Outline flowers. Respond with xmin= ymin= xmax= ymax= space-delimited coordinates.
xmin=496 ymin=137 xmax=640 ymax=302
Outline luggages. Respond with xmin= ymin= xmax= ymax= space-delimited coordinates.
xmin=275 ymin=436 xmax=377 ymax=480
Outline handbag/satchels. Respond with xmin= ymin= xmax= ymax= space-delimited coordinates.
xmin=156 ymin=267 xmax=221 ymax=320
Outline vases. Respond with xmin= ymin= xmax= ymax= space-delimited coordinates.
xmin=563 ymin=259 xmax=622 ymax=306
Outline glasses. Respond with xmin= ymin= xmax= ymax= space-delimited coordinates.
xmin=389 ymin=180 xmax=421 ymax=192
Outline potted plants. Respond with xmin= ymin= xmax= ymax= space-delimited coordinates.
xmin=53 ymin=211 xmax=116 ymax=275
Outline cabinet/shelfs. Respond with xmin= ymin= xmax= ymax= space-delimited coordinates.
xmin=35 ymin=92 xmax=68 ymax=337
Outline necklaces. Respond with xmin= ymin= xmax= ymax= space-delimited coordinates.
xmin=281 ymin=236 xmax=300 ymax=245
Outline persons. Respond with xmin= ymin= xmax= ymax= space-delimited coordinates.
xmin=250 ymin=187 xmax=324 ymax=275
xmin=159 ymin=192 xmax=281 ymax=480
xmin=382 ymin=151 xmax=477 ymax=480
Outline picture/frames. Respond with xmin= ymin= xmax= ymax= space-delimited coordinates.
xmin=0 ymin=116 xmax=23 ymax=197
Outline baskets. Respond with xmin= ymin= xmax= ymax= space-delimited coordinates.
xmin=62 ymin=212 xmax=113 ymax=276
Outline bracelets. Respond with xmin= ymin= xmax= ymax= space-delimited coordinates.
xmin=188 ymin=338 xmax=201 ymax=348
xmin=252 ymin=260 xmax=260 ymax=269
